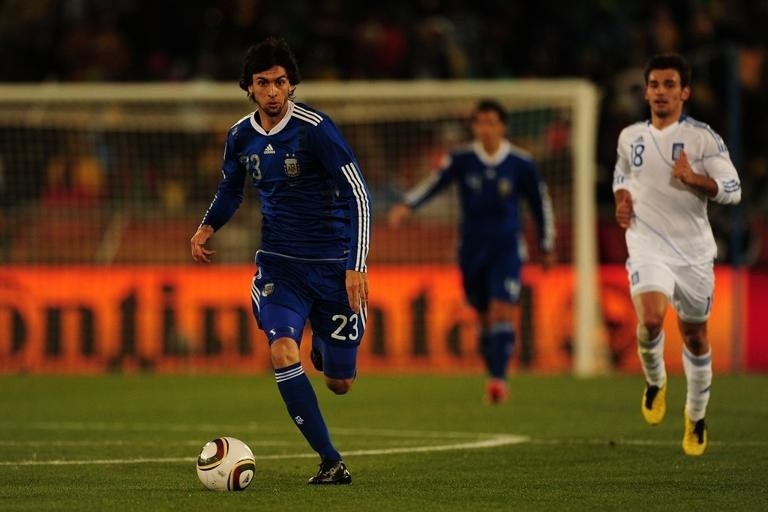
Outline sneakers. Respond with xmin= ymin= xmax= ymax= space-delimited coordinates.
xmin=486 ymin=382 xmax=507 ymax=404
xmin=308 ymin=459 xmax=351 ymax=484
xmin=681 ymin=406 xmax=707 ymax=456
xmin=641 ymin=372 xmax=666 ymax=425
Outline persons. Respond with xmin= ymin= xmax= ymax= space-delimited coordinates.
xmin=188 ymin=41 xmax=372 ymax=483
xmin=385 ymin=97 xmax=558 ymax=407
xmin=1 ymin=1 xmax=767 ymax=273
xmin=609 ymin=53 xmax=744 ymax=455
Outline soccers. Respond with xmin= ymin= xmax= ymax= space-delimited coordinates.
xmin=196 ymin=438 xmax=256 ymax=492
